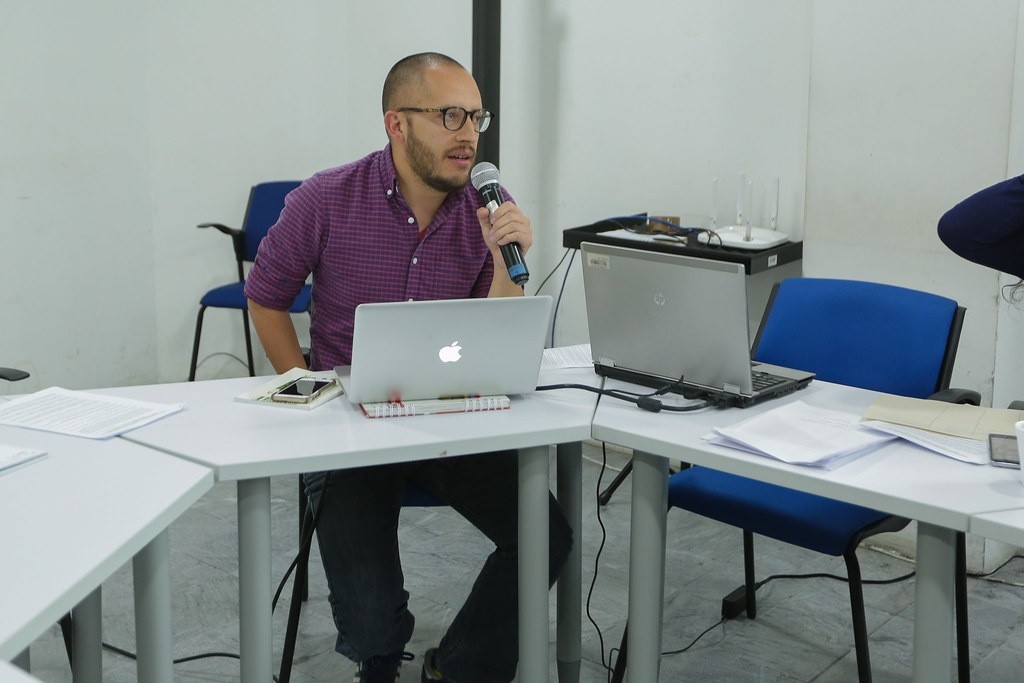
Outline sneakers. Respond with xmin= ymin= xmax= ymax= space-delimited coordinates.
xmin=356 ymin=645 xmax=415 ymax=683
xmin=422 ymin=647 xmax=449 ymax=683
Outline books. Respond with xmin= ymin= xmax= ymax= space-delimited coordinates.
xmin=361 ymin=394 xmax=510 ymax=419
xmin=0 ymin=447 xmax=48 ymax=476
xmin=235 ymin=366 xmax=344 ymax=410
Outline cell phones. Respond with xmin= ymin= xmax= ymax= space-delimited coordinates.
xmin=271 ymin=377 xmax=337 ymax=404
xmin=988 ymin=434 xmax=1021 ymax=470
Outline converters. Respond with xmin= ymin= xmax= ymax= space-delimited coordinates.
xmin=721 ymin=584 xmax=747 ymax=620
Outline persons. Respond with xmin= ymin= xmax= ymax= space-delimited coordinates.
xmin=243 ymin=52 xmax=574 ymax=683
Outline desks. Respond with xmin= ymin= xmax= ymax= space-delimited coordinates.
xmin=71 ymin=371 xmax=603 ymax=683
xmin=561 ymin=210 xmax=803 ymax=506
xmin=590 ymin=378 xmax=1024 ymax=683
xmin=0 ymin=397 xmax=214 ymax=683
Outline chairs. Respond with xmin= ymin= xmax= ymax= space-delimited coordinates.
xmin=611 ymin=279 xmax=982 ymax=682
xmin=187 ymin=180 xmax=313 ymax=381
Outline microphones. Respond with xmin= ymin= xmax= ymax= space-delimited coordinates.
xmin=470 ymin=161 xmax=529 ymax=286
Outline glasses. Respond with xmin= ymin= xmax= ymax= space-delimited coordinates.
xmin=396 ymin=107 xmax=496 ymax=134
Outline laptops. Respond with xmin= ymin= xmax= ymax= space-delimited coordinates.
xmin=334 ymin=296 xmax=553 ymax=404
xmin=581 ymin=241 xmax=816 ymax=409
